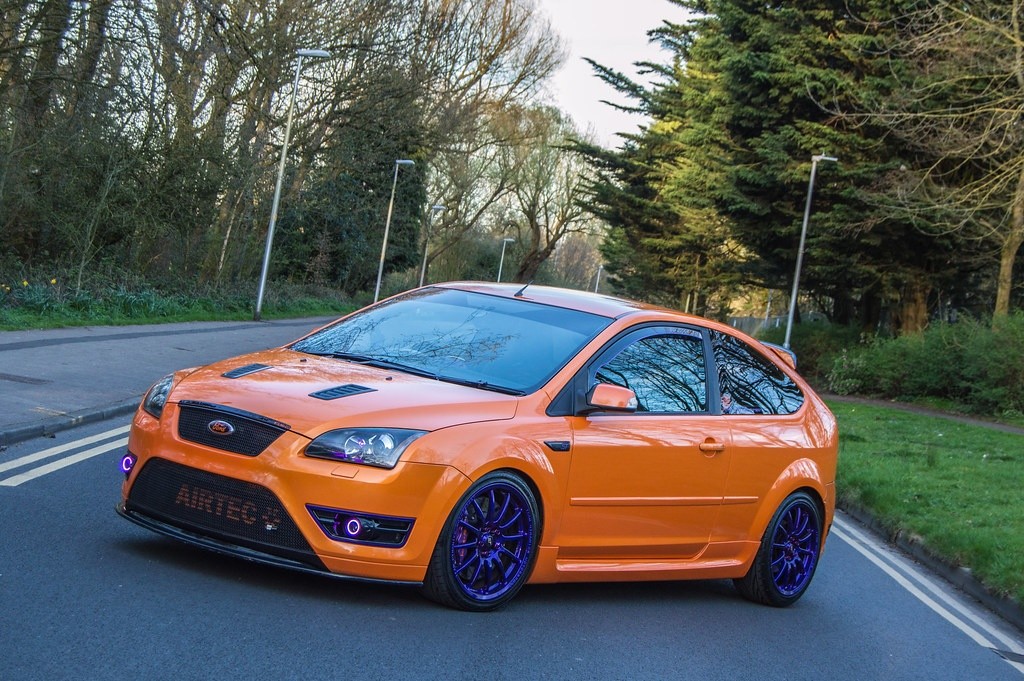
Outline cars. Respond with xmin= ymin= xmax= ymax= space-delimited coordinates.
xmin=111 ymin=280 xmax=841 ymax=617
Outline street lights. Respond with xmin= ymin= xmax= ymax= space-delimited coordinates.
xmin=420 ymin=205 xmax=447 ymax=289
xmin=497 ymin=237 xmax=516 ymax=283
xmin=780 ymin=153 xmax=839 ymax=351
xmin=253 ymin=48 xmax=334 ymax=322
xmin=594 ymin=265 xmax=604 ymax=292
xmin=372 ymin=159 xmax=416 ymax=306
